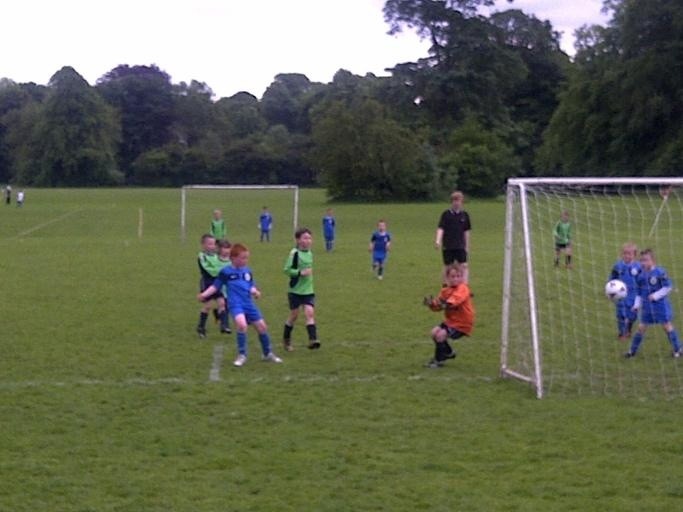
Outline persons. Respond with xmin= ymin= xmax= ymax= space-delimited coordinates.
xmin=193 ymin=204 xmax=337 ymax=367
xmin=434 ymin=190 xmax=474 ymax=298
xmin=551 ymin=209 xmax=575 ymax=269
xmin=422 ymin=264 xmax=476 ymax=368
xmin=367 ymin=218 xmax=392 ymax=281
xmin=16 ymin=190 xmax=26 ymax=208
xmin=5 ymin=182 xmax=13 ymax=205
xmin=623 ymin=247 xmax=683 ymax=359
xmin=608 ymin=243 xmax=639 ymax=341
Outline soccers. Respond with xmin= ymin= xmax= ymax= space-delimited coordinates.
xmin=604 ymin=279 xmax=628 ymax=302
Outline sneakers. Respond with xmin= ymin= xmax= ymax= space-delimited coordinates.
xmin=672 ymin=348 xmax=683 ymax=358
xmin=197 ymin=327 xmax=206 ymax=339
xmin=284 ymin=343 xmax=294 ymax=352
xmin=444 ymin=349 xmax=457 ymax=360
xmin=308 ymin=340 xmax=321 ymax=350
xmin=233 ymin=354 xmax=248 ymax=367
xmin=221 ymin=326 xmax=233 ymax=334
xmin=423 ymin=358 xmax=443 ymax=369
xmin=261 ymin=352 xmax=283 ymax=364
xmin=623 ymin=351 xmax=635 ymax=359
